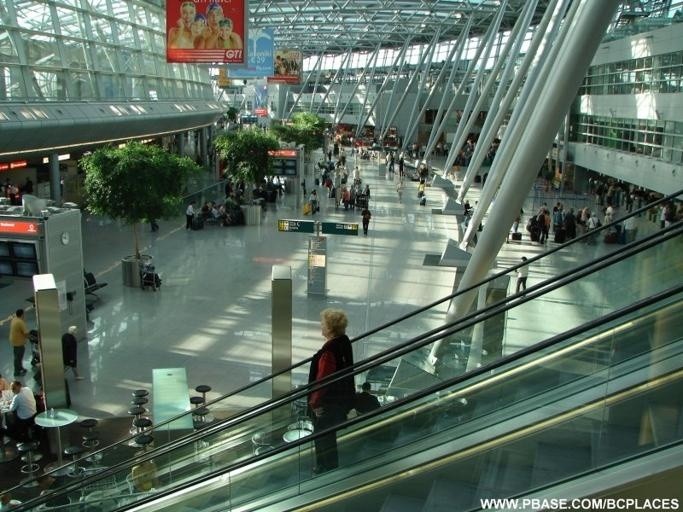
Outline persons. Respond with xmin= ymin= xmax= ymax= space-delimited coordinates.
xmin=3 ymin=380 xmax=37 ymax=442
xmin=215 ymin=16 xmax=242 ymax=49
xmin=280 ymin=57 xmax=290 ymax=70
xmin=432 ymin=137 xmax=504 ymax=172
xmin=192 ymin=1 xmax=225 ymax=49
xmin=167 ymin=0 xmax=198 ymax=49
xmin=190 ymin=12 xmax=206 ymax=37
xmin=513 ymin=255 xmax=529 ymax=299
xmin=301 ymin=118 xmax=432 ymax=237
xmin=305 ymin=305 xmax=357 ymax=476
xmin=510 ymin=168 xmax=681 ymax=246
xmin=9 ymin=184 xmax=17 ymax=205
xmin=149 ymin=218 xmax=159 ymax=233
xmin=8 ymin=307 xmax=32 ymax=379
xmin=184 ymin=173 xmax=286 ymax=229
xmin=23 ymin=176 xmax=34 ymax=194
xmin=354 ymin=380 xmax=381 ymax=416
xmin=275 ymin=55 xmax=285 ymax=73
xmin=60 ymin=176 xmax=64 ymax=185
xmin=0 ymin=373 xmax=6 ymax=401
xmin=141 ymin=265 xmax=161 ymax=288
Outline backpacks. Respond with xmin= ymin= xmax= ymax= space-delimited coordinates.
xmin=596 ymin=218 xmax=601 ymax=228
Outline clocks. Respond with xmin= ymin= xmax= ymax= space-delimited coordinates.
xmin=61 ymin=231 xmax=70 ymax=245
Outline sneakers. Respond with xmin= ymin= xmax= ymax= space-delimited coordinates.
xmin=14 ymin=369 xmax=27 ymax=376
xmin=75 ymin=376 xmax=86 ymax=380
xmin=364 ymin=232 xmax=367 ymax=235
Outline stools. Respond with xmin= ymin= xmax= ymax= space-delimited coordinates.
xmin=0 ymin=385 xmax=312 ymax=512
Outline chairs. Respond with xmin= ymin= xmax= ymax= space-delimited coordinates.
xmin=141 ymin=269 xmax=160 ymax=292
xmin=83 ymin=268 xmax=108 ymax=301
xmin=25 ymin=297 xmax=35 ymax=302
xmin=354 ymin=410 xmax=384 ymax=440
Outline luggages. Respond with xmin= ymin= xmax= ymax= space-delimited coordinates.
xmin=531 ymin=225 xmax=541 ymax=242
xmin=525 ymin=215 xmax=538 ymax=232
xmin=420 ymin=194 xmax=426 ymax=206
xmin=554 ymin=228 xmax=566 ymax=244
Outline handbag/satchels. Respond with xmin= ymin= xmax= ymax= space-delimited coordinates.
xmin=317 ymin=202 xmax=320 ymax=211
xmin=365 ymin=214 xmax=371 ymax=220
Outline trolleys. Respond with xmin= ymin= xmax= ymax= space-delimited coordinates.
xmin=29 ymin=329 xmax=41 ymax=367
